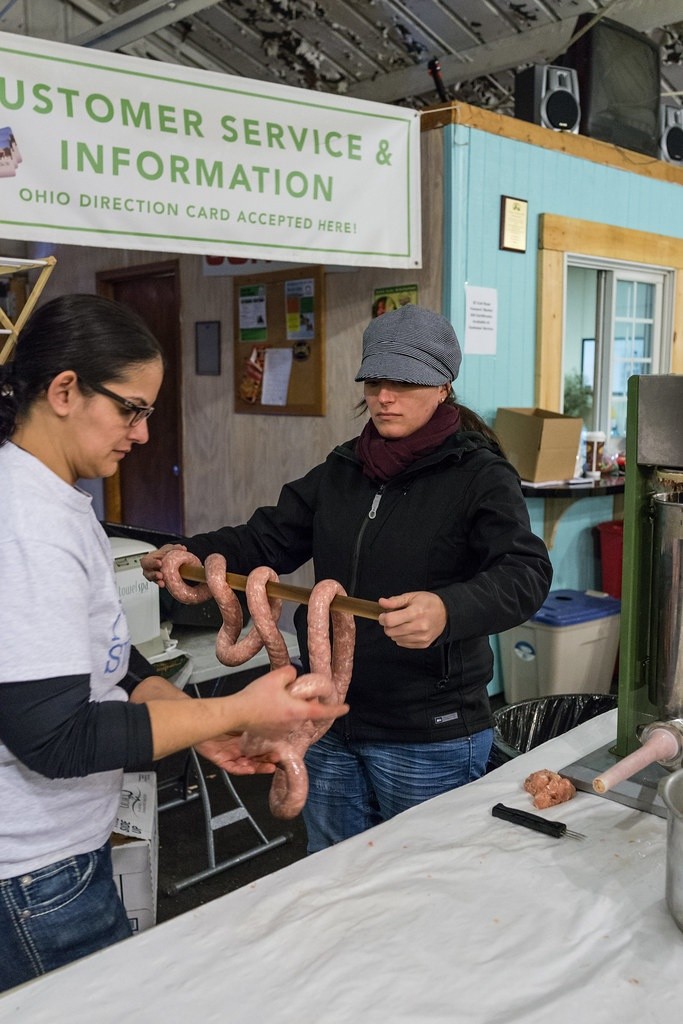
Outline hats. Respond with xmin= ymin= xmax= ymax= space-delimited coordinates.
xmin=354 ymin=305 xmax=461 ymax=386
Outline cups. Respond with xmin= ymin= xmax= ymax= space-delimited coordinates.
xmin=585 ymin=431 xmax=606 ymax=471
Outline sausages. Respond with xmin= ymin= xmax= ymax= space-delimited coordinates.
xmin=160 ymin=550 xmax=355 ymax=820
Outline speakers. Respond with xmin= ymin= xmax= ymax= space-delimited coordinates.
xmin=515 ymin=64 xmax=580 ymax=134
xmin=657 ymin=103 xmax=683 ymax=167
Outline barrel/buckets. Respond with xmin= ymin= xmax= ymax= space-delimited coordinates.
xmin=641 ymin=492 xmax=683 ymax=721
xmin=597 ymin=521 xmax=625 ymax=602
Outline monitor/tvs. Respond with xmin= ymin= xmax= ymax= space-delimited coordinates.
xmin=551 ymin=12 xmax=663 ymax=159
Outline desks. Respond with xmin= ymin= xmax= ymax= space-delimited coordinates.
xmin=0 ymin=698 xmax=683 ymax=1024
xmin=157 ymin=626 xmax=302 ymax=888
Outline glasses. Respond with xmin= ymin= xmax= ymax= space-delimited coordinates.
xmin=78 ymin=375 xmax=154 ymax=427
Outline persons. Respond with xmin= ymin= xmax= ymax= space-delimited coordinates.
xmin=140 ymin=306 xmax=552 ymax=859
xmin=0 ymin=295 xmax=351 ymax=990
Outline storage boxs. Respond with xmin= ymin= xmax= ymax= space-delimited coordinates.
xmin=495 ymin=587 xmax=623 ymax=697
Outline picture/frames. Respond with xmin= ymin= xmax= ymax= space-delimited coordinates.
xmin=194 ymin=320 xmax=223 ymax=378
xmin=499 ymin=194 xmax=530 ymax=254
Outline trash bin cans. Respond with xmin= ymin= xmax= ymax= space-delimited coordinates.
xmin=488 ymin=694 xmax=618 ymax=770
